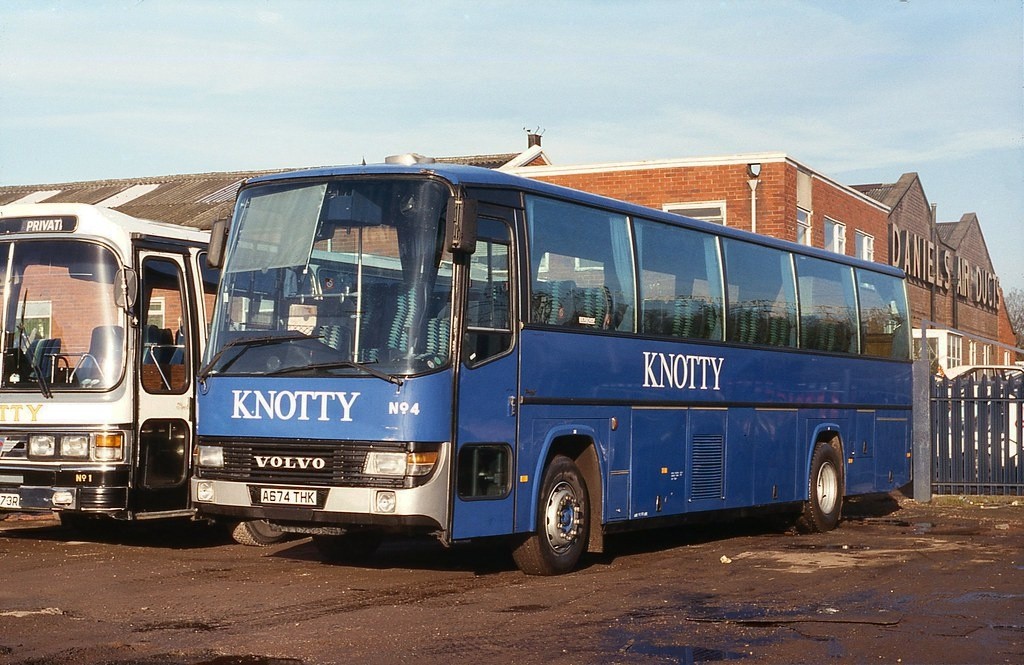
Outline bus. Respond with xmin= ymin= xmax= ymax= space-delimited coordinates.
xmin=0 ymin=202 xmax=543 ymax=547
xmin=192 ymin=153 xmax=922 ymax=578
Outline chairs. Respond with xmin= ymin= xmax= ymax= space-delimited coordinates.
xmin=313 ymin=283 xmax=856 ymax=364
xmin=76 ymin=323 xmax=184 ymax=387
xmin=23 ymin=337 xmax=62 ymax=382
